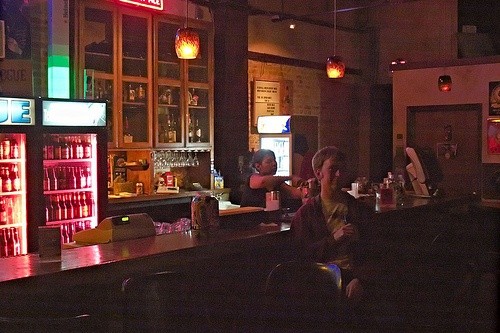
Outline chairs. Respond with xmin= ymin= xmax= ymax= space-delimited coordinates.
xmin=0 ymin=221 xmax=474 ymax=333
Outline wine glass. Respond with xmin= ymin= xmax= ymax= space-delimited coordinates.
xmin=154 ymin=150 xmax=199 ymax=167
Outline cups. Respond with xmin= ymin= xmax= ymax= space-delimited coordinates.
xmin=351 ymin=183 xmax=358 ymax=195
xmin=154 ymin=218 xmax=191 ymax=235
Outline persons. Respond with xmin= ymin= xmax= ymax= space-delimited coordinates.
xmin=241 ymin=150 xmax=317 ymax=208
xmin=290 ymin=146 xmax=389 ymax=333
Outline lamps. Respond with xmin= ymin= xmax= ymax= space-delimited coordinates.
xmin=174 ymin=0 xmax=200 ymax=59
xmin=325 ymin=0 xmax=346 ymax=78
xmin=438 ymin=73 xmax=453 ymax=93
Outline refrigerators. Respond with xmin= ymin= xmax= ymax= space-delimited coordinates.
xmin=34 ymin=97 xmax=108 ymax=251
xmin=0 ymin=93 xmax=38 ymax=259
xmin=257 ymin=115 xmax=319 ymax=186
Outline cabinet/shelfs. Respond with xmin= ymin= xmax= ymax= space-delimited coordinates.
xmin=71 ymin=0 xmax=216 ymax=202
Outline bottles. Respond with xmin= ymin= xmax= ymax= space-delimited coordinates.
xmin=43 ymin=136 xmax=94 ymax=244
xmin=385 ymin=175 xmax=405 ymax=188
xmin=87 ymin=72 xmax=202 ymax=143
xmin=0 ymin=137 xmax=20 ymax=259
